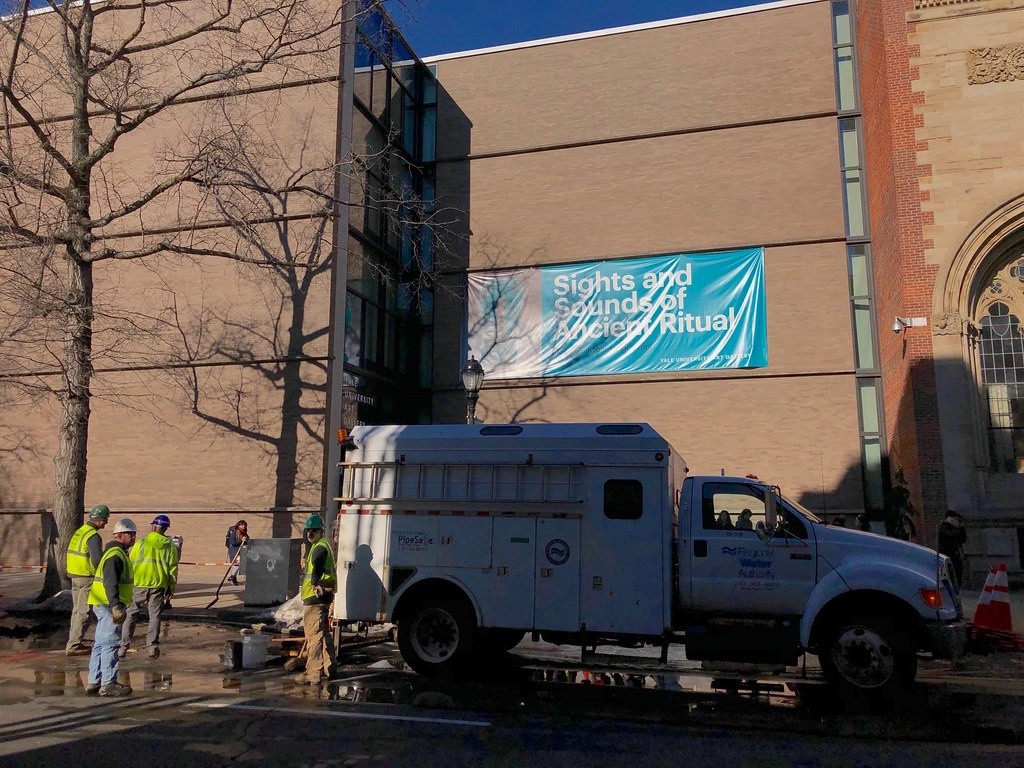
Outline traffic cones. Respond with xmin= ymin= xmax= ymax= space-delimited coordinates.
xmin=977 ymin=561 xmax=1020 ymax=636
xmin=968 ymin=566 xmax=997 ymax=625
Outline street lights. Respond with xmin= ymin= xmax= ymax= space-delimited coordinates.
xmin=461 ymin=355 xmax=485 ymax=425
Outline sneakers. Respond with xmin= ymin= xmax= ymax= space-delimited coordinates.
xmin=86 ymin=684 xmax=101 ymax=694
xmin=229 ymin=575 xmax=240 ymax=586
xmin=292 ymin=670 xmax=322 ymax=686
xmin=99 ymin=682 xmax=132 ymax=698
xmin=66 ymin=644 xmax=91 ymax=656
xmin=149 ymin=646 xmax=160 ymax=656
xmin=326 ymin=663 xmax=338 ymax=680
xmin=118 ymin=648 xmax=124 ymax=656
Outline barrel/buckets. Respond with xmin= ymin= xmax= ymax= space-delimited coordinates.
xmin=242 ymin=635 xmax=269 ymax=669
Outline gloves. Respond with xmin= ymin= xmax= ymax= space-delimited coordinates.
xmin=113 ymin=605 xmax=127 ymax=625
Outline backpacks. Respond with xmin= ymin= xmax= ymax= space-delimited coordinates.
xmin=226 ymin=527 xmax=234 ymax=547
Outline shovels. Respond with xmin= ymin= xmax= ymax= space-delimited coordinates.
xmin=204 ymin=538 xmax=247 ymax=609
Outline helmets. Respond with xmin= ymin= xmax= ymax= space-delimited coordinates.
xmin=89 ymin=505 xmax=110 ymax=518
xmin=150 ymin=515 xmax=171 ymax=527
xmin=305 ymin=514 xmax=325 ymax=529
xmin=113 ymin=518 xmax=137 ymax=534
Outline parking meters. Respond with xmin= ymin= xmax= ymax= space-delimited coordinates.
xmin=163 ymin=535 xmax=183 ymax=610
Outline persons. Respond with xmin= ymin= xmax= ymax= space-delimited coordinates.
xmin=227 ymin=520 xmax=249 ymax=585
xmin=937 ymin=509 xmax=967 ymax=590
xmin=295 ymin=515 xmax=336 ymax=685
xmin=535 ymin=669 xmax=682 ymax=691
xmin=66 ymin=505 xmax=110 ymax=656
xmin=118 ymin=515 xmax=178 ymax=657
xmin=716 ymin=511 xmax=734 ymax=529
xmin=85 ymin=518 xmax=132 ymax=696
xmin=736 ymin=509 xmax=752 ymax=529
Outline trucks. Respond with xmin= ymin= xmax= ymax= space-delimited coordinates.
xmin=327 ymin=423 xmax=976 ymax=702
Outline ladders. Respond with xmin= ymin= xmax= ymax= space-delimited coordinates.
xmin=332 ymin=460 xmax=587 ymax=506
